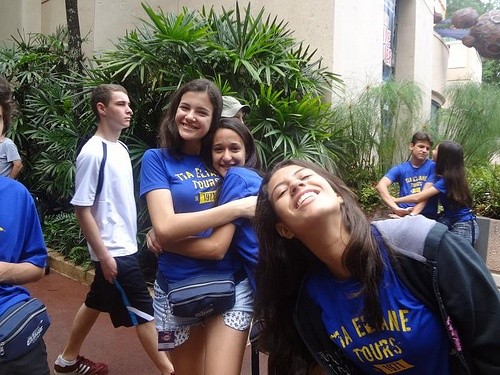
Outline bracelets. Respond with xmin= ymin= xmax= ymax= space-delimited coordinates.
xmin=7 ymin=175 xmax=10 ymax=178
xmin=408 ymin=214 xmax=414 ymax=216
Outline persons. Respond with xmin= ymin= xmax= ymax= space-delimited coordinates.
xmin=146 ymin=118 xmax=328 ymax=375
xmin=254 ymin=159 xmax=500 ymax=375
xmin=220 ymin=95 xmax=244 ymax=125
xmin=138 ymin=79 xmax=260 ymax=375
xmin=52 ymin=83 xmax=176 ymax=375
xmin=0 ymin=74 xmax=51 ymax=375
xmin=376 ymin=131 xmax=481 ymax=248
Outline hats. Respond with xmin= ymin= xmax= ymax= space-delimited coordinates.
xmin=220 ymin=95 xmax=251 ymax=118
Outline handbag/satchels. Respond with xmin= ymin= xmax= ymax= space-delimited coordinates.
xmin=437 ymin=211 xmax=453 ymax=231
xmin=248 ymin=316 xmax=272 ymax=375
xmin=0 ymin=295 xmax=52 ymax=364
xmin=166 ymin=270 xmax=236 ymax=317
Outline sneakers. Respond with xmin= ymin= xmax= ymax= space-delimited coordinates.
xmin=53 ymin=353 xmax=109 ymax=375
